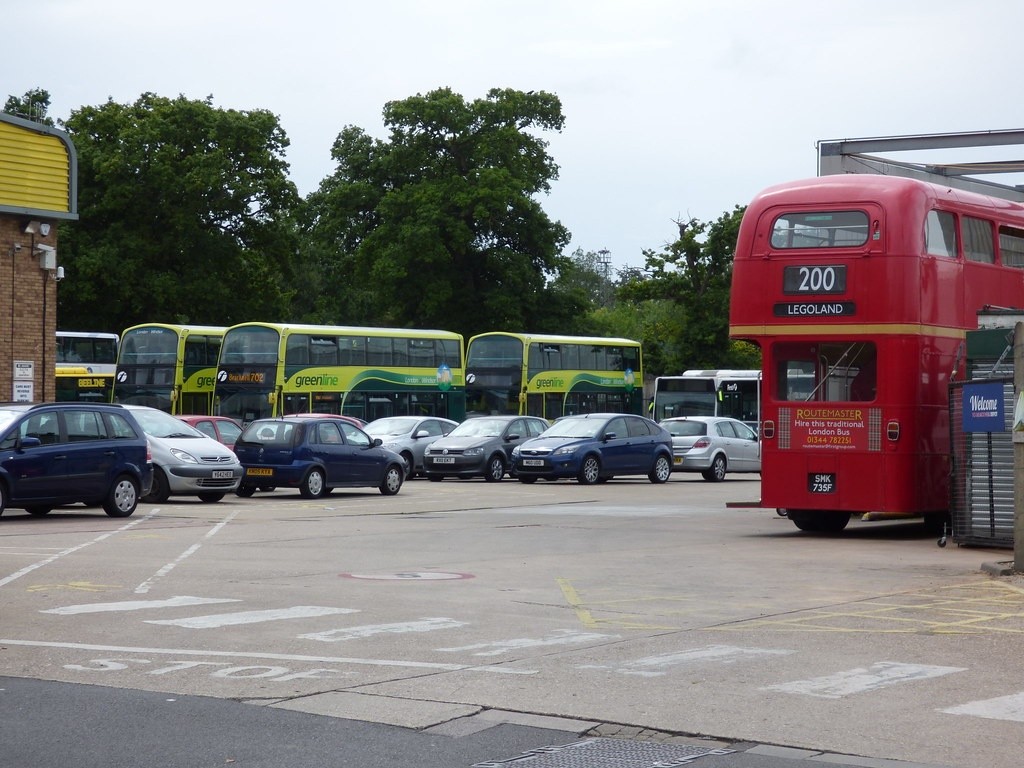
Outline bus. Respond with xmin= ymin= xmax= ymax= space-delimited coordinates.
xmin=733 ymin=175 xmax=1023 ymax=532
xmin=55 ymin=332 xmax=120 ymax=401
xmin=216 ymin=322 xmax=464 ymax=422
xmin=654 ymin=371 xmax=765 ymax=420
xmin=112 ymin=324 xmax=226 ymax=417
xmin=466 ymin=333 xmax=644 ymax=414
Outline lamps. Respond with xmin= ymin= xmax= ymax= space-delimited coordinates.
xmin=50 ymin=266 xmax=65 ymax=282
xmin=22 ymin=219 xmax=40 ymax=234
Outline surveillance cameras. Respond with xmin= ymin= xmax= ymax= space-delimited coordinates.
xmin=13 ymin=242 xmax=22 ymax=253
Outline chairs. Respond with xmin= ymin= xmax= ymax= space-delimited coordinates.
xmin=261 ymin=428 xmax=274 ymax=440
xmin=313 ymin=429 xmax=329 ymax=443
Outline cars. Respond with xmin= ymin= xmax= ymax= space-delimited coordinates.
xmin=512 ymin=417 xmax=674 ymax=487
xmin=1 ymin=404 xmax=152 ymax=518
xmin=237 ymin=419 xmax=406 ymax=496
xmin=120 ymin=402 xmax=242 ymax=506
xmin=425 ymin=415 xmax=548 ymax=484
xmin=365 ymin=415 xmax=459 ymax=479
xmin=659 ymin=419 xmax=760 ymax=483
